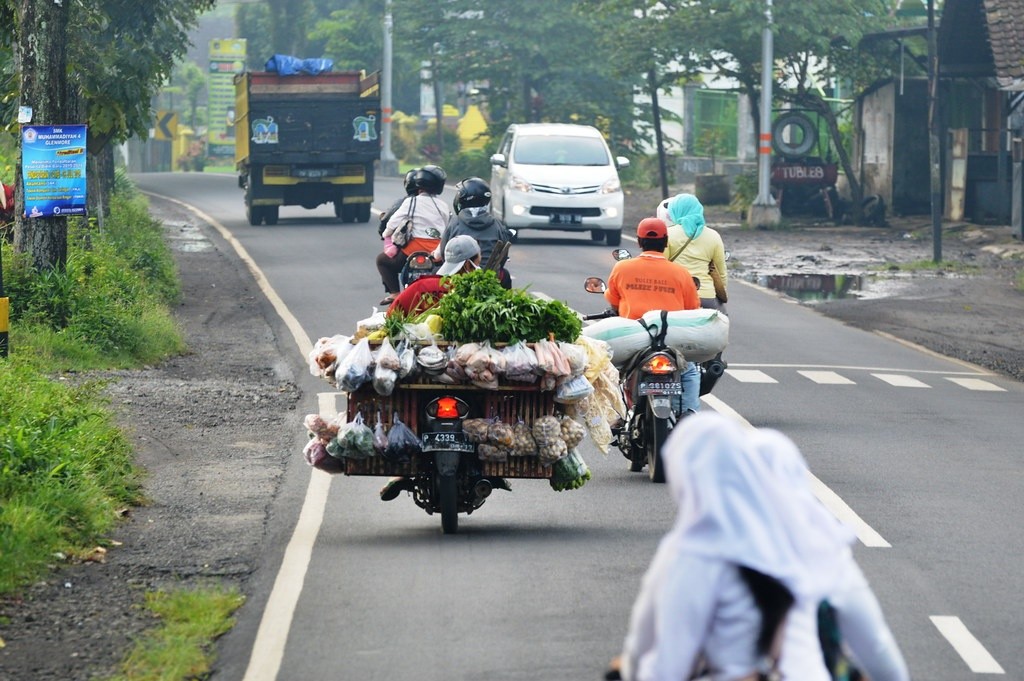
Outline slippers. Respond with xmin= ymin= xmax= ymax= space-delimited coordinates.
xmin=380 ymin=479 xmax=412 ymax=501
xmin=491 ymin=478 xmax=512 ymax=492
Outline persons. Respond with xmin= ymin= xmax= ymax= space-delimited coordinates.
xmin=605 ymin=217 xmax=701 ymax=412
xmin=376 ymin=164 xmax=513 ymax=289
xmin=613 ymin=250 xmax=619 ymax=259
xmin=614 ymin=413 xmax=910 ymax=681
xmin=586 ymin=278 xmax=603 ymax=293
xmin=661 ymin=193 xmax=730 ymax=361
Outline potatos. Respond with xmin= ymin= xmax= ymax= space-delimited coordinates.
xmin=462 ymin=417 xmax=583 ymax=464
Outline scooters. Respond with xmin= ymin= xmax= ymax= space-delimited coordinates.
xmin=397 ymin=395 xmax=502 ymax=536
xmin=378 ymin=213 xmax=436 ymax=289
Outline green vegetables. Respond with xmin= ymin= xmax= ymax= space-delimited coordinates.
xmin=380 ymin=269 xmax=582 ymax=343
xmin=550 ymin=452 xmax=591 ymax=492
xmin=328 ymin=430 xmax=372 ymax=459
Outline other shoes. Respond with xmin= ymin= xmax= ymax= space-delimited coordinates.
xmin=611 ymin=417 xmax=626 ymax=429
xmin=379 ymin=297 xmax=394 ymax=305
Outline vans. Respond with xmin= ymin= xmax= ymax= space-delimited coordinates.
xmin=489 ymin=124 xmax=629 ymax=247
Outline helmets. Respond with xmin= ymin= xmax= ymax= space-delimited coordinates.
xmin=452 ymin=178 xmax=492 ymax=215
xmin=404 ymin=168 xmax=420 ymax=195
xmin=656 ymin=196 xmax=677 ymax=227
xmin=416 ymin=165 xmax=447 ymax=194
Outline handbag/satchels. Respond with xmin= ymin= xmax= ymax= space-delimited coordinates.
xmin=391 ymin=196 xmax=417 ymax=247
xmin=384 ymin=236 xmax=397 ymax=258
xmin=301 ymin=306 xmax=628 ymax=482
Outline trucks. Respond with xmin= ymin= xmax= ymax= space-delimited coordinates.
xmin=227 ymin=70 xmax=381 ymax=226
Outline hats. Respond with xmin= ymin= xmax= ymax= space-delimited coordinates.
xmin=436 ymin=234 xmax=480 ymax=275
xmin=637 ymin=218 xmax=668 ymax=238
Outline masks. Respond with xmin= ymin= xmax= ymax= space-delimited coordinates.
xmin=463 ymin=259 xmax=481 ymax=274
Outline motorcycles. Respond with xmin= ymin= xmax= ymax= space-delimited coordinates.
xmin=612 ymin=248 xmax=725 ymax=398
xmin=582 ymin=276 xmax=684 ymax=483
